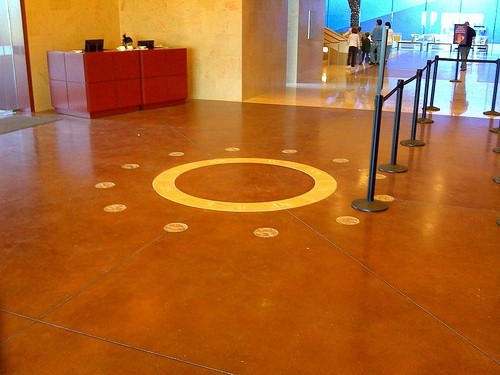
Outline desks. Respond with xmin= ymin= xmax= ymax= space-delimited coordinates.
xmin=427 ymin=43 xmax=452 ymax=52
xmin=47 ymin=47 xmax=187 ymax=120
xmin=472 ymin=44 xmax=489 ymax=55
xmin=397 ymin=41 xmax=423 ymax=51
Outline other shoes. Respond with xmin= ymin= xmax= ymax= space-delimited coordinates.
xmin=347 ymin=65 xmax=350 ymax=68
xmin=350 ymin=66 xmax=354 ymax=68
xmin=369 ymin=63 xmax=373 ymax=64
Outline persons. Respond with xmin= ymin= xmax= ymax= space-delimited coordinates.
xmin=458 ymin=21 xmax=476 ymax=71
xmin=121 ymin=34 xmax=133 ymax=48
xmin=346 ymin=19 xmax=393 ymax=69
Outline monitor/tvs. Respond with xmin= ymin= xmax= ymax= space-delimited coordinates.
xmin=138 ymin=40 xmax=154 ymax=49
xmin=85 ymin=39 xmax=103 ymax=52
